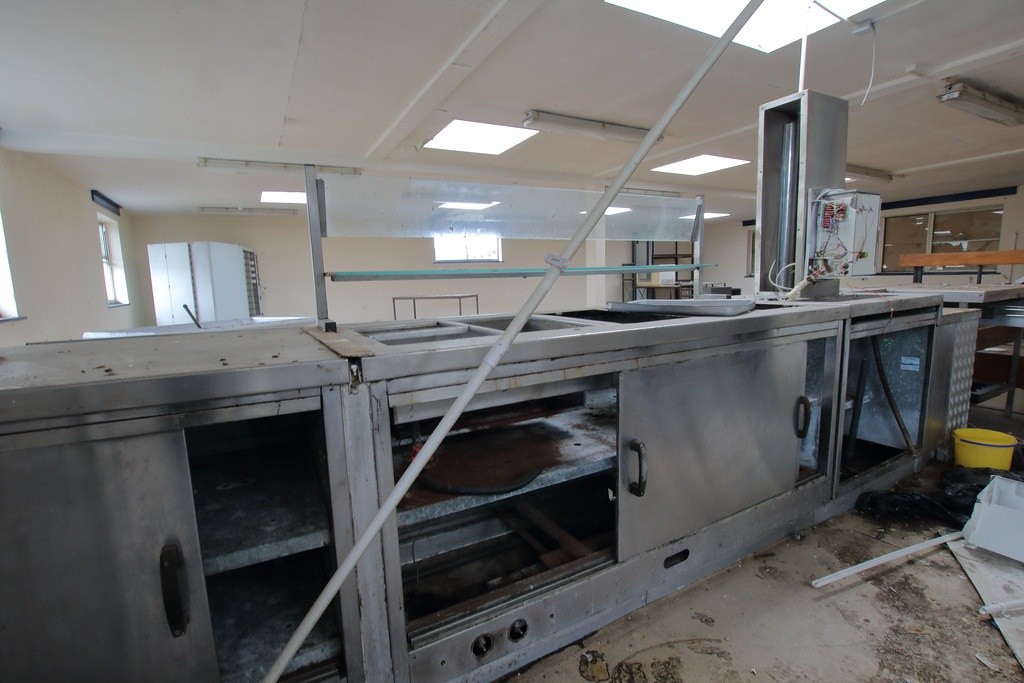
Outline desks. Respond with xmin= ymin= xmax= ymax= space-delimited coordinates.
xmin=635 ymin=284 xmax=681 ymax=299
xmin=887 ymin=285 xmax=1024 ymax=308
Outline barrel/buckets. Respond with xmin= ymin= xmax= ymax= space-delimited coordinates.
xmin=954 ymin=429 xmax=1018 ymax=471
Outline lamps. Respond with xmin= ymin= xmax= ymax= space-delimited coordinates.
xmin=520 ymin=109 xmax=664 ymax=146
xmin=200 ymin=206 xmax=299 ymax=217
xmin=934 ymin=83 xmax=1024 ymax=128
xmin=846 ymin=164 xmax=893 ymax=184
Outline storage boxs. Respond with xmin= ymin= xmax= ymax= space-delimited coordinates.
xmin=650 ymin=264 xmax=676 ymax=300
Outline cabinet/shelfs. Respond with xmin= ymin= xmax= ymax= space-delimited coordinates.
xmin=148 ymin=241 xmax=262 ymax=326
xmin=392 ymin=294 xmax=480 ymax=320
xmin=0 ymin=305 xmax=938 ymax=683
